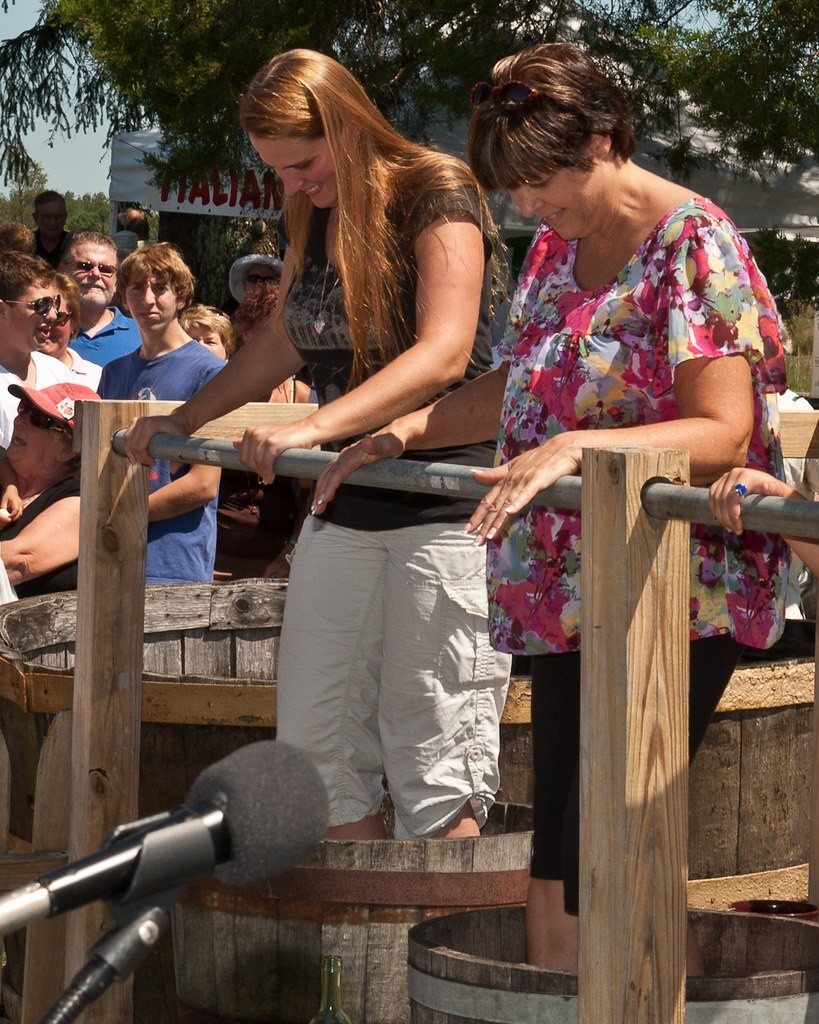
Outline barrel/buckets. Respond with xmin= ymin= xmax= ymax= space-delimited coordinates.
xmin=407 ymin=904 xmax=819 ymax=1024
xmin=171 ymin=792 xmax=536 ymax=1024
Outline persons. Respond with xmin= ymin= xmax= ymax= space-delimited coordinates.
xmin=310 ymin=45 xmax=790 ymax=975
xmin=121 ymin=48 xmax=507 ymax=839
xmin=707 ymin=415 xmax=818 ymax=580
xmin=0 ymin=190 xmax=310 ymax=604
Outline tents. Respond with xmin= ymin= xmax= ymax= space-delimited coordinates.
xmin=108 ymin=0 xmax=819 ymax=239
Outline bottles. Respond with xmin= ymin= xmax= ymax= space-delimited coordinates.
xmin=308 ymin=955 xmax=354 ymax=1024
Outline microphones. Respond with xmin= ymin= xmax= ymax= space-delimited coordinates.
xmin=0 ymin=740 xmax=326 ymax=942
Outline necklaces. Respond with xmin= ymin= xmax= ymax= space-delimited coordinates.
xmin=314 ymin=259 xmax=339 ymax=334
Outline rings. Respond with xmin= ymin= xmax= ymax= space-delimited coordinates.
xmin=732 ymin=484 xmax=747 ymax=496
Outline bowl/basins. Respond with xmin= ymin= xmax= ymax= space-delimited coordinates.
xmin=731 ymin=899 xmax=819 ymax=922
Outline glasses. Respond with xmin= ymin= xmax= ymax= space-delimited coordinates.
xmin=246 ymin=273 xmax=276 ymax=287
xmin=64 ymin=262 xmax=119 ymax=274
xmin=54 ymin=310 xmax=74 ymax=327
xmin=16 ymin=398 xmax=75 ymax=444
xmin=4 ymin=293 xmax=61 ymax=316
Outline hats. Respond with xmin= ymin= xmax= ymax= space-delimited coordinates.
xmin=7 ymin=381 xmax=103 ymax=431
xmin=228 ymin=225 xmax=282 ymax=304
